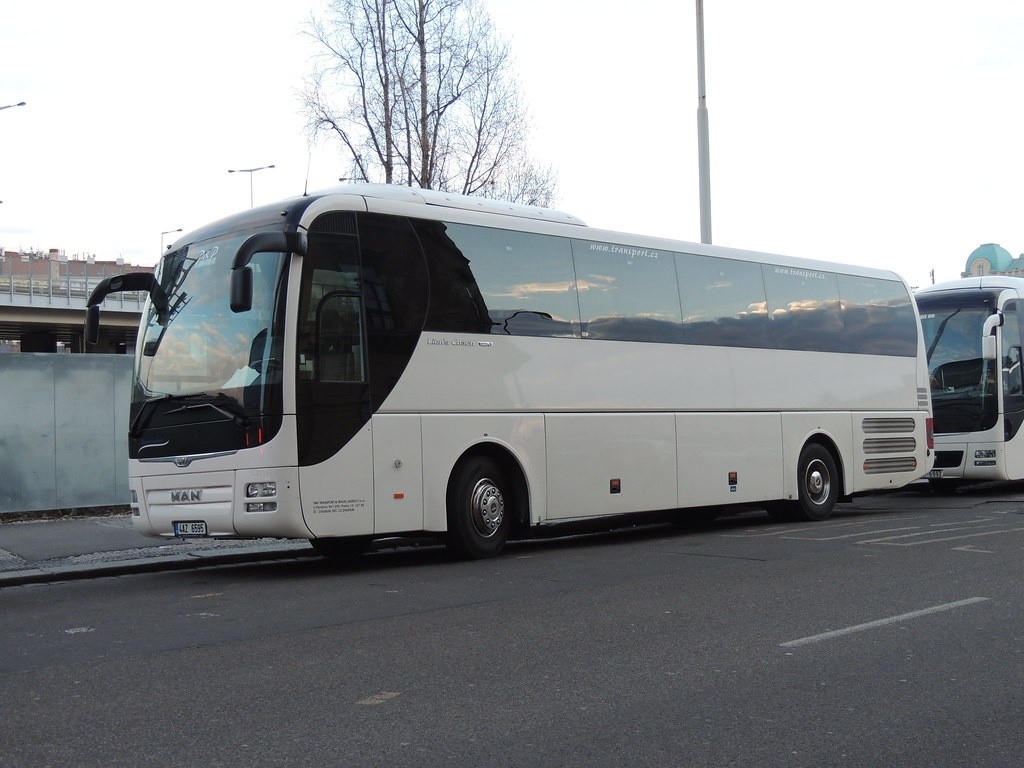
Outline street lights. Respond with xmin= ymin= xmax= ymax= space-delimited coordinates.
xmin=228 ymin=165 xmax=276 ymax=209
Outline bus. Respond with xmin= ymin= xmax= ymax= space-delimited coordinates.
xmin=910 ymin=275 xmax=1024 ymax=491
xmin=84 ymin=182 xmax=937 ymax=564
